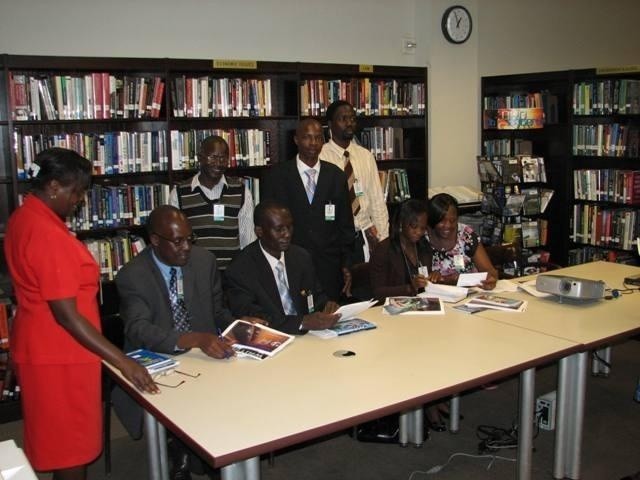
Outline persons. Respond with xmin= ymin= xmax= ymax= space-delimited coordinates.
xmin=114 ymin=205 xmax=270 ymax=480
xmin=223 ymin=197 xmax=342 ymax=335
xmin=366 ymin=199 xmax=464 ymax=434
xmin=168 ymin=136 xmax=258 ymax=312
xmin=318 ymin=100 xmax=390 ymax=263
xmin=3 ymin=146 xmax=159 ymax=480
xmin=428 ymin=192 xmax=497 ymax=294
xmin=260 ymin=118 xmax=356 ymax=307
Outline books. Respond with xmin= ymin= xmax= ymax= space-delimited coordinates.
xmin=217 ymin=320 xmax=295 ymax=361
xmin=309 ymin=318 xmax=376 ymax=338
xmin=299 ymin=77 xmax=426 ymax=201
xmin=13 ymin=121 xmax=170 ymax=179
xmin=124 ymin=348 xmax=178 ymax=373
xmin=171 ymin=128 xmax=271 ymax=171
xmin=8 ymin=71 xmax=164 ymax=121
xmin=479 ymin=79 xmax=637 ymax=287
xmin=82 ymin=230 xmax=148 ymax=281
xmin=174 ymin=176 xmax=260 ymax=207
xmin=17 ymin=179 xmax=170 ymax=232
xmin=169 ymin=75 xmax=273 ymax=118
xmin=383 ymin=296 xmax=444 ymax=315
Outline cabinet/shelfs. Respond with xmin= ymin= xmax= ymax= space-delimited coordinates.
xmin=1 ymin=54 xmax=298 ymax=423
xmin=560 ymin=72 xmax=640 ymax=269
xmin=296 ymin=65 xmax=428 ymax=213
xmin=481 ymin=69 xmax=597 ymax=270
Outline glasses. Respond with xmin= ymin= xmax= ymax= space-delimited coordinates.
xmin=151 ymin=232 xmax=198 ymax=247
xmin=196 ymin=152 xmax=228 ymax=165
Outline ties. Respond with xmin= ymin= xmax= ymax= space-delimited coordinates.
xmin=304 ymin=169 xmax=317 ymax=206
xmin=168 ymin=268 xmax=193 ymax=334
xmin=276 ymin=260 xmax=298 ymax=316
xmin=344 ymin=150 xmax=360 ymax=216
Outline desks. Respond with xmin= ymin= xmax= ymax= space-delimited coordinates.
xmin=101 ymin=298 xmax=583 ymax=480
xmin=427 ymin=260 xmax=640 ymax=480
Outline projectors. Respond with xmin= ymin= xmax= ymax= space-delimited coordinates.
xmin=536 ymin=275 xmax=606 ymax=304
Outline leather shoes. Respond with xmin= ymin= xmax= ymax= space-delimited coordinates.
xmin=168 ymin=440 xmax=190 ymax=480
xmin=437 ymin=401 xmax=463 ymax=420
xmin=357 ymin=426 xmax=399 ymax=442
xmin=428 ymin=414 xmax=446 ymax=431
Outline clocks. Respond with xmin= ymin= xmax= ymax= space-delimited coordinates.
xmin=441 ymin=5 xmax=472 ymax=44
